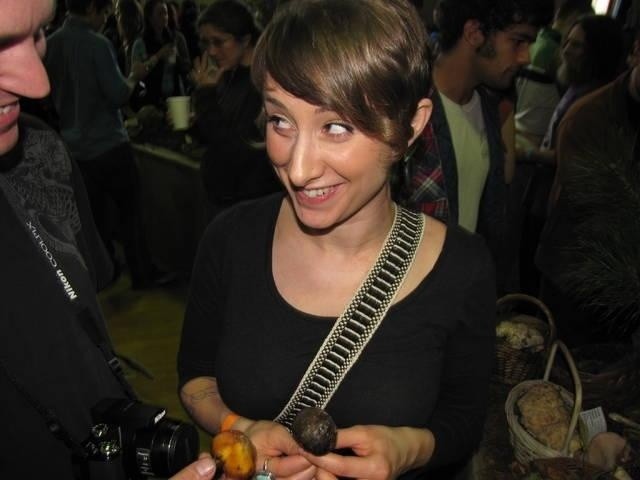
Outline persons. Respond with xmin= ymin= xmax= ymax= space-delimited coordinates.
xmin=15 ymin=0 xmax=639 ymax=440
xmin=0 ymin=0 xmax=215 ymax=480
xmin=178 ymin=1 xmax=501 ymax=480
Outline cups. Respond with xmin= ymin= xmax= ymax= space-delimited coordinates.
xmin=165 ymin=94 xmax=193 ymax=131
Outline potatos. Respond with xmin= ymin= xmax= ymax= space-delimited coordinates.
xmin=210 ymin=426 xmax=258 ymax=480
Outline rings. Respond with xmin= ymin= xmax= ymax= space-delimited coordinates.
xmin=254 ymin=457 xmax=274 ymax=480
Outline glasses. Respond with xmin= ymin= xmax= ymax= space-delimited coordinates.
xmin=197 ymin=35 xmax=234 ymax=53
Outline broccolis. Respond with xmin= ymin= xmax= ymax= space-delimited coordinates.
xmin=496 ymin=320 xmax=547 ymax=355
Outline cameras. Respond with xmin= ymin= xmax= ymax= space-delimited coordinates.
xmin=66 ymin=394 xmax=200 ymax=480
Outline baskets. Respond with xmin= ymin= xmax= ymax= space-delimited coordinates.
xmin=505 ymin=339 xmax=587 ymax=467
xmin=489 ymin=295 xmax=557 ymax=386
